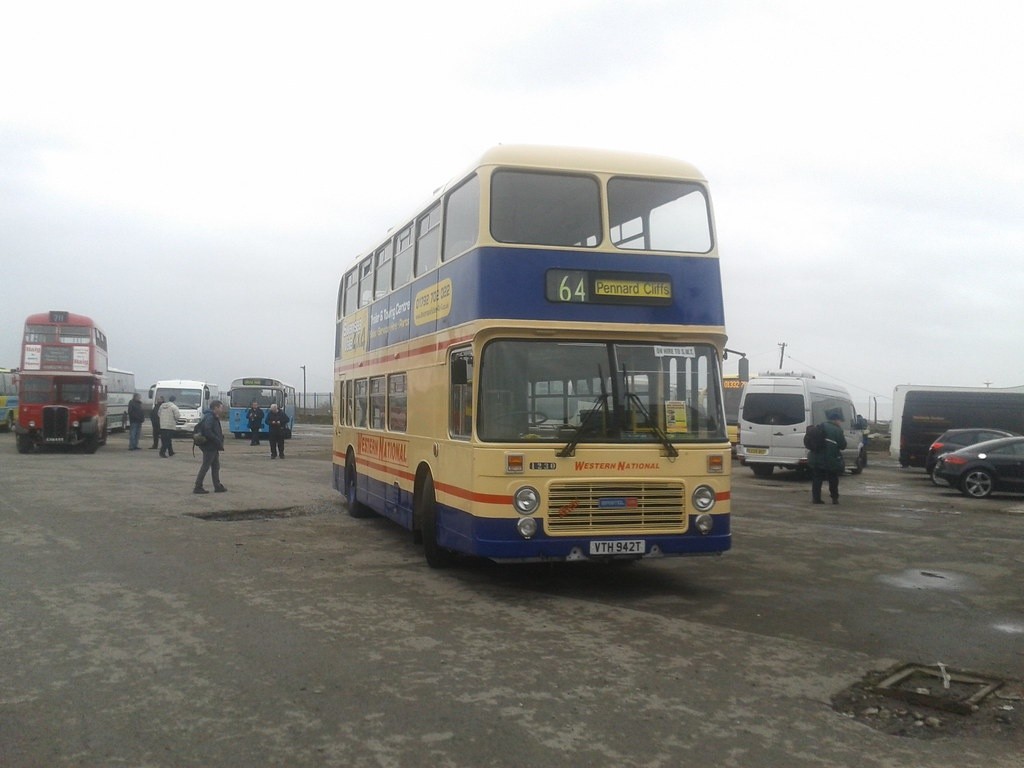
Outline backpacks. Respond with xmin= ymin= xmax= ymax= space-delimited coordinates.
xmin=804 ymin=423 xmax=825 ymax=451
xmin=191 ymin=415 xmax=208 ymax=451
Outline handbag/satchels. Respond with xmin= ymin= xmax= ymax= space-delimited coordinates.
xmin=280 ymin=423 xmax=291 ymax=439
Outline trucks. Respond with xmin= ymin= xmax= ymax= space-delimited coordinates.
xmin=736 ymin=369 xmax=869 ymax=475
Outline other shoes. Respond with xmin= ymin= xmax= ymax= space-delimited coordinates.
xmin=813 ymin=501 xmax=825 ymax=504
xmin=250 ymin=442 xmax=260 ymax=446
xmin=832 ymin=500 xmax=839 ymax=504
xmin=271 ymin=454 xmax=277 ymax=459
xmin=279 ymin=454 xmax=284 ymax=459
xmin=193 ymin=488 xmax=209 ymax=493
xmin=214 ymin=486 xmax=228 ymax=492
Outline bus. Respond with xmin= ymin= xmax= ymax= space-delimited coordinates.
xmin=227 ymin=377 xmax=296 ymax=438
xmin=0 ymin=368 xmax=18 ymax=432
xmin=889 ymin=385 xmax=1024 ymax=467
xmin=331 ymin=142 xmax=749 ymax=570
xmin=699 ymin=373 xmax=754 ymax=458
xmin=149 ymin=380 xmax=220 ymax=436
xmin=15 ymin=311 xmax=107 ymax=454
xmin=103 ymin=367 xmax=135 ymax=433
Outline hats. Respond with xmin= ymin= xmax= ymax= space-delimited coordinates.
xmin=825 ymin=408 xmax=843 ymax=419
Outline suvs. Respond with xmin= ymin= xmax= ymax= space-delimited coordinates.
xmin=927 ymin=427 xmax=1024 ymax=487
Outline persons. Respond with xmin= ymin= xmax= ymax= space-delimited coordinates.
xmin=128 ymin=393 xmax=145 ymax=450
xmin=246 ymin=402 xmax=265 ymax=446
xmin=192 ymin=400 xmax=228 ymax=494
xmin=148 ymin=395 xmax=165 ymax=449
xmin=157 ymin=395 xmax=180 ymax=458
xmin=265 ymin=404 xmax=290 ymax=459
xmin=807 ymin=410 xmax=847 ymax=504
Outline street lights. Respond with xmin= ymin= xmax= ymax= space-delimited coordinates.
xmin=300 ymin=365 xmax=305 ymax=410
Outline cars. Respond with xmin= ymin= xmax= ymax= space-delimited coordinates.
xmin=933 ymin=436 xmax=1024 ymax=498
